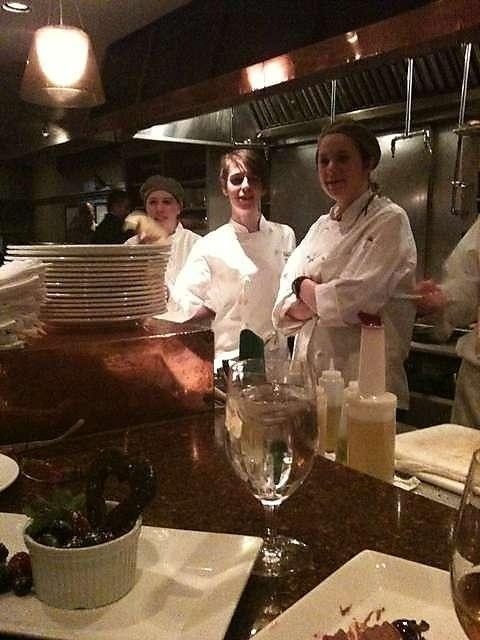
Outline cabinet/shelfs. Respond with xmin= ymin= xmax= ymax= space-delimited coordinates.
xmin=1 ymin=317 xmax=214 ymax=440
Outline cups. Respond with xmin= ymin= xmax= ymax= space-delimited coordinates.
xmin=225 ymin=358 xmax=327 ymax=578
xmin=448 ymin=446 xmax=480 ymax=638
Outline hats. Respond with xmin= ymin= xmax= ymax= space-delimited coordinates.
xmin=139 ymin=175 xmax=184 ymax=205
xmin=319 ymin=117 xmax=381 ymax=172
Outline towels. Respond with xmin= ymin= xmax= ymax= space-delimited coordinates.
xmin=393 ymin=423 xmax=480 ymax=495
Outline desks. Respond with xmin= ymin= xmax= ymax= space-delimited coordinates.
xmin=396 ymin=323 xmax=472 ymax=431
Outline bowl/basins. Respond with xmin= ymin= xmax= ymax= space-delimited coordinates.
xmin=20 ymin=499 xmax=143 ymax=609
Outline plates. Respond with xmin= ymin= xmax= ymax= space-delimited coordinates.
xmin=3 ymin=243 xmax=174 ymax=332
xmin=1 ymin=509 xmax=264 ymax=640
xmin=0 ymin=444 xmax=20 ymax=495
xmin=246 ymin=519 xmax=480 ymax=639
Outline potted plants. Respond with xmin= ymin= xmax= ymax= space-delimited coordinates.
xmin=15 ymin=440 xmax=157 ymax=610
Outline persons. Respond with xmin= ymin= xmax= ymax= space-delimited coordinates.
xmin=170 ymin=146 xmax=288 ymax=376
xmin=69 ymin=200 xmax=98 ymax=244
xmin=122 ymin=175 xmax=203 ymax=322
xmin=408 ymin=213 xmax=480 ymax=428
xmin=91 ymin=188 xmax=137 ymax=245
xmin=270 ymin=117 xmax=412 ymax=424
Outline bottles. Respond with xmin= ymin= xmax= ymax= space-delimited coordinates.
xmin=347 ymin=311 xmax=397 ymax=484
xmin=316 ymin=386 xmax=327 ymax=457
xmin=319 ymin=358 xmax=345 ymax=452
xmin=335 ymin=380 xmax=358 ymax=466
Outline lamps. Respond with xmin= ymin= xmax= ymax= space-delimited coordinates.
xmin=3 ymin=0 xmax=29 ymax=12
xmin=19 ymin=4 xmax=103 ymax=111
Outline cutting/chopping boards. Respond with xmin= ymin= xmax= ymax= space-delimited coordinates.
xmin=395 ymin=424 xmax=480 ymax=476
